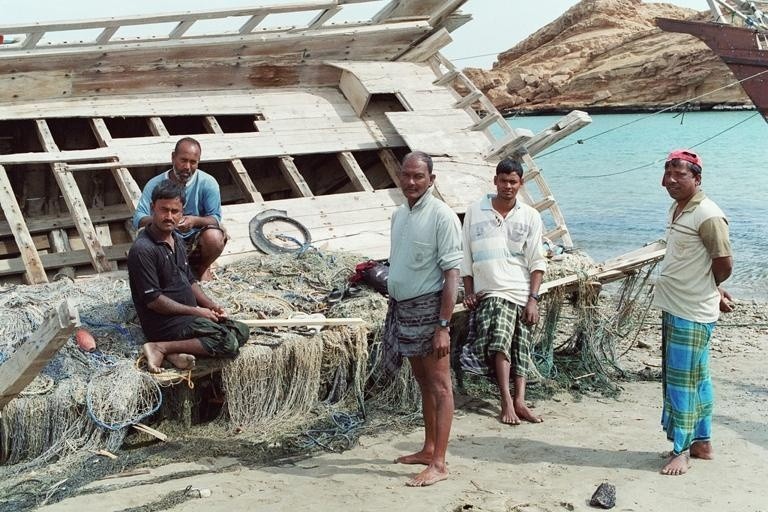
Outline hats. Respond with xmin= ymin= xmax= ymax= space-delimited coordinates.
xmin=661 ymin=150 xmax=703 ymax=188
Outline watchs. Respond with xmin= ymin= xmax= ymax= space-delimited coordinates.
xmin=437 ymin=319 xmax=451 ymax=328
xmin=529 ymin=293 xmax=539 ymax=300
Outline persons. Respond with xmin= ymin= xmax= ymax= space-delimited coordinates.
xmin=132 ymin=137 xmax=231 ymax=282
xmin=462 ymin=157 xmax=548 ymax=427
xmin=653 ymin=148 xmax=738 ymax=476
xmin=384 ymin=149 xmax=464 ymax=488
xmin=127 ymin=178 xmax=250 ymax=375
